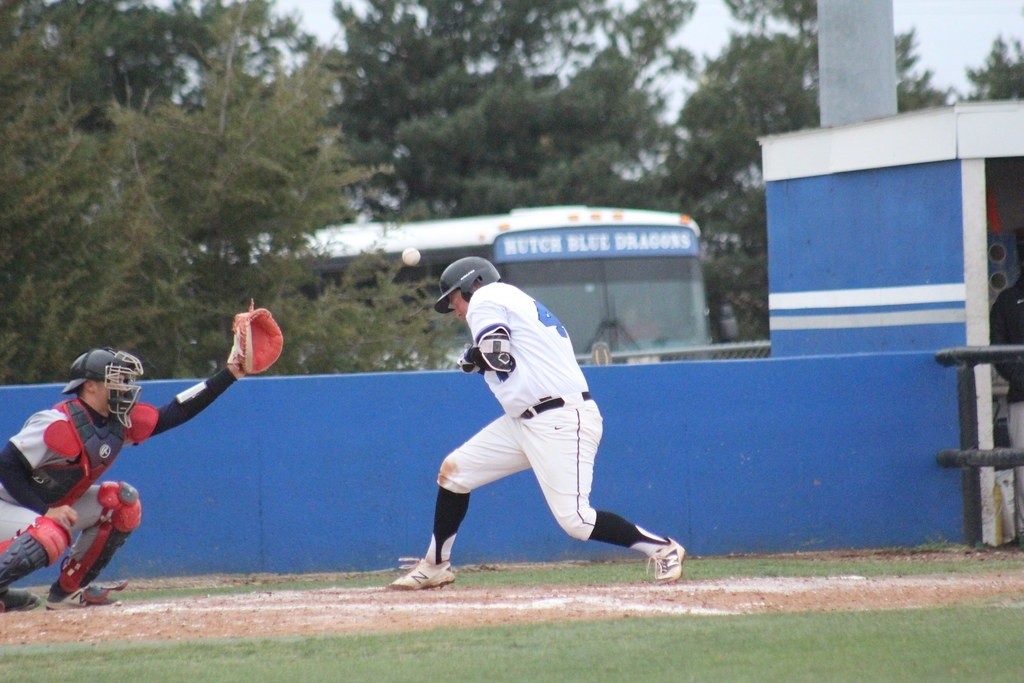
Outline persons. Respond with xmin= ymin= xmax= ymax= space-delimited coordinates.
xmin=0 ymin=307 xmax=284 ymax=615
xmin=388 ymin=256 xmax=685 ymax=589
xmin=988 ymin=261 xmax=1023 ymax=551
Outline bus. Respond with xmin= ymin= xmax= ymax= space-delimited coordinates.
xmin=189 ymin=206 xmax=739 ymax=371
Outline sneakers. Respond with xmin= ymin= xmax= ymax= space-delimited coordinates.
xmin=647 ymin=536 xmax=685 ymax=584
xmin=0 ymin=588 xmax=41 ymax=612
xmin=388 ymin=557 xmax=455 ymax=590
xmin=46 ymin=581 xmax=122 ymax=609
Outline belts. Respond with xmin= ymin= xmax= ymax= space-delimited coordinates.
xmin=521 ymin=391 xmax=592 ymax=419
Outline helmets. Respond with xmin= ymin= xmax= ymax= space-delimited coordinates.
xmin=434 ymin=256 xmax=501 ymax=313
xmin=62 ymin=348 xmax=130 ymax=393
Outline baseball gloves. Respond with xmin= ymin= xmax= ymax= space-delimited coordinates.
xmin=226 ymin=296 xmax=284 ymax=382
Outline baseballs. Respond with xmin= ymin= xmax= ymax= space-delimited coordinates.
xmin=402 ymin=247 xmax=421 ymax=266
